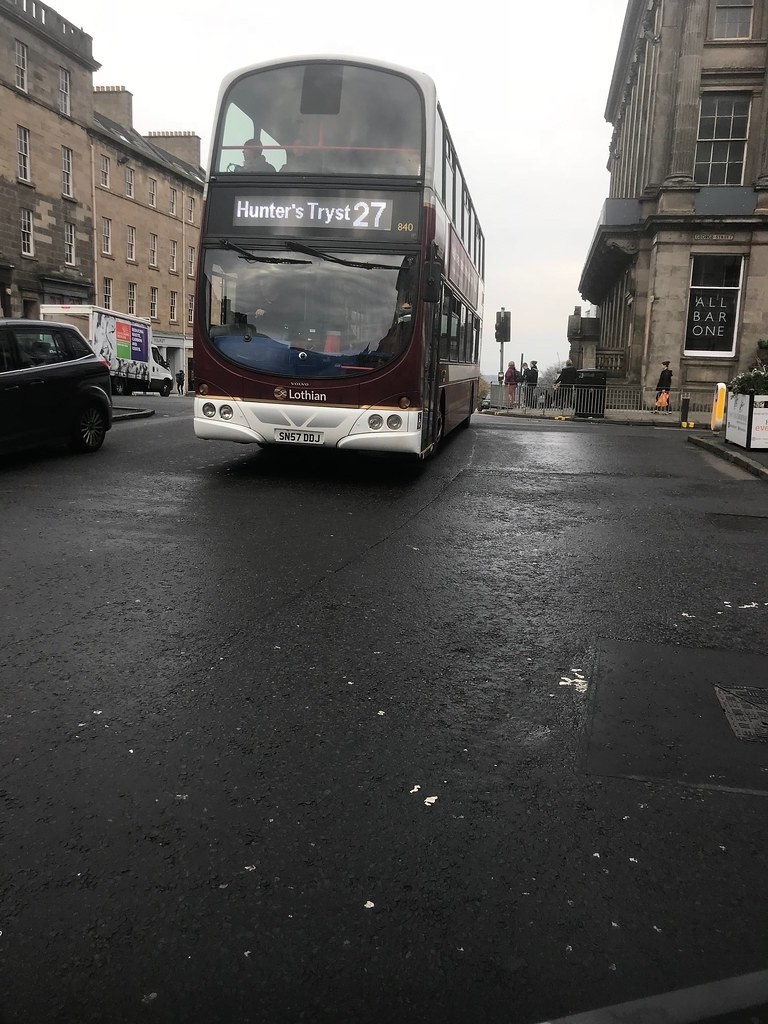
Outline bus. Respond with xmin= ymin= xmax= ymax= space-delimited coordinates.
xmin=192 ymin=56 xmax=486 ymax=460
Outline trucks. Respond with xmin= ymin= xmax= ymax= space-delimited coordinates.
xmin=40 ymin=304 xmax=174 ymax=397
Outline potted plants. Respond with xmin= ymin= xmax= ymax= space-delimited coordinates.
xmin=725 ymin=368 xmax=768 ymax=451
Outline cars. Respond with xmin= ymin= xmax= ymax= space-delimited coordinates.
xmin=0 ymin=320 xmax=113 ymax=453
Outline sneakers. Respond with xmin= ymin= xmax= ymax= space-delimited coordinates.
xmin=664 ymin=410 xmax=672 ymax=415
xmin=650 ymin=410 xmax=659 ymax=414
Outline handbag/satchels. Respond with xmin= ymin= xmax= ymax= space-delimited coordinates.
xmin=655 ymin=391 xmax=669 ymax=407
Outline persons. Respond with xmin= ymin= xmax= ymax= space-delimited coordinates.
xmin=651 ymin=361 xmax=673 ymax=415
xmin=235 ymin=137 xmax=319 ymax=174
xmin=177 ymin=370 xmax=184 ymax=396
xmin=504 ymin=359 xmax=579 ymax=410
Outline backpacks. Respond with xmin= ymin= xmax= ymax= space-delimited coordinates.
xmin=511 ymin=368 xmax=523 ymax=383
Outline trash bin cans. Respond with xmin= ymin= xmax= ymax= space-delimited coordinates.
xmin=575 ymin=368 xmax=607 ymax=418
